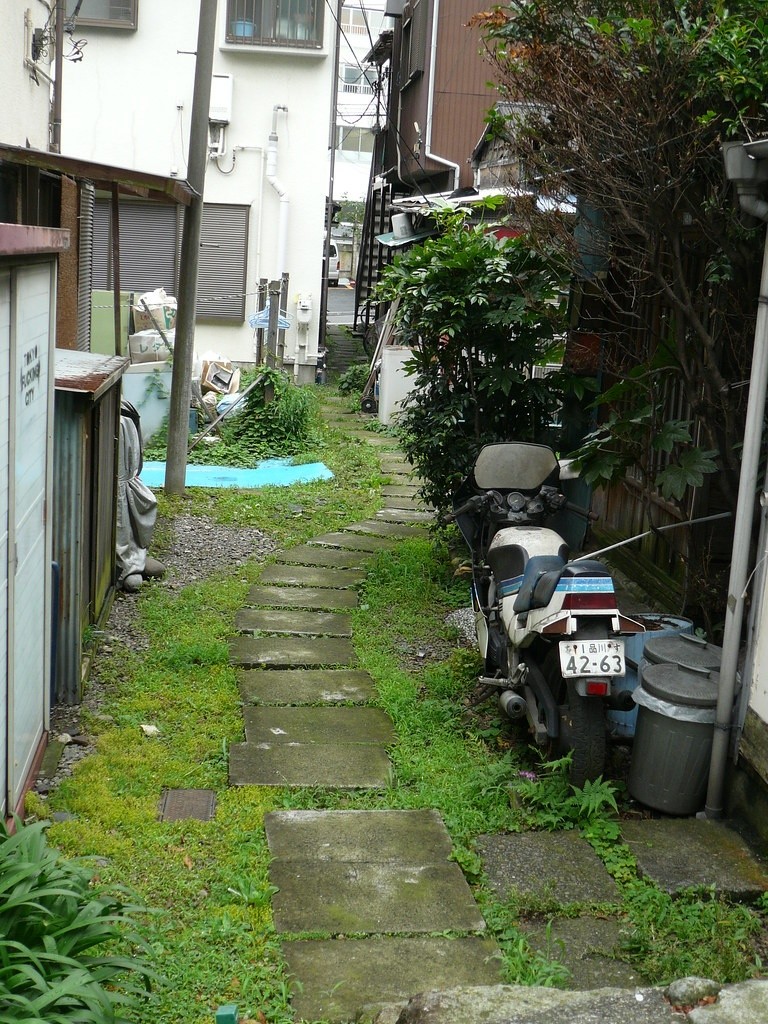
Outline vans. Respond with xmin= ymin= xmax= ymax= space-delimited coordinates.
xmin=323 ymin=238 xmax=341 ymax=287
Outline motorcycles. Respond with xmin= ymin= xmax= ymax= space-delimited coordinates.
xmin=428 ymin=439 xmax=639 ymax=798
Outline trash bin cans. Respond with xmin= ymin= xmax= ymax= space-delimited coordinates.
xmin=625 ymin=633 xmax=742 ymax=815
